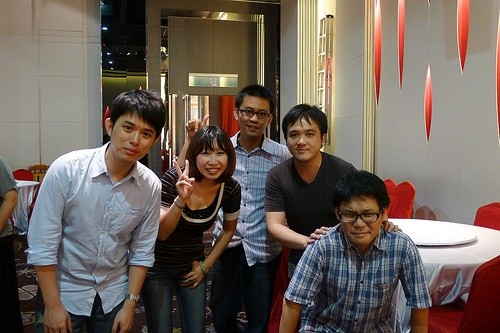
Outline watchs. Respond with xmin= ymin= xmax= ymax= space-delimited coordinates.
xmin=125 ymin=294 xmax=140 ymax=305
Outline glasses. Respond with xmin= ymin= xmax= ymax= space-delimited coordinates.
xmin=239 ymin=108 xmax=269 ymax=120
xmin=338 ymin=204 xmax=383 ymax=224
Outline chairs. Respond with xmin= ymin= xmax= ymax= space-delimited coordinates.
xmin=13 ymin=169 xmax=33 ymax=181
xmin=381 ymin=177 xmax=500 ymax=333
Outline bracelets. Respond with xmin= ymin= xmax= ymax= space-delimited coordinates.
xmin=200 ymin=260 xmax=209 ymax=275
xmin=174 ymin=196 xmax=184 ymax=209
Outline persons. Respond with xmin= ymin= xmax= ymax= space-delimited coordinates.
xmin=0 ymin=157 xmax=25 ymax=333
xmin=139 ymin=124 xmax=242 ymax=333
xmin=176 ymin=85 xmax=292 ymax=333
xmin=27 ymin=89 xmax=165 ymax=333
xmin=277 ymin=170 xmax=433 ymax=333
xmin=266 ymin=103 xmax=401 ymax=333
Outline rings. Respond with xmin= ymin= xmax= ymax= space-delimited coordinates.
xmin=187 ymin=278 xmax=195 ymax=285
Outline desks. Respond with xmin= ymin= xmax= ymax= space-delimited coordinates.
xmin=386 ymin=218 xmax=500 ymax=333
xmin=12 ymin=180 xmax=40 ymax=235
